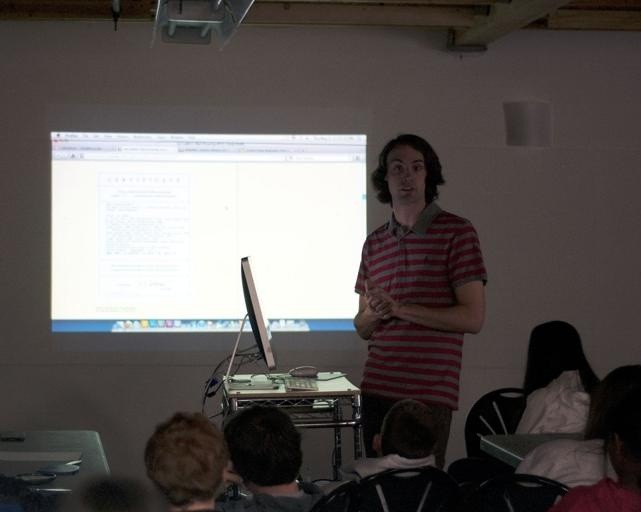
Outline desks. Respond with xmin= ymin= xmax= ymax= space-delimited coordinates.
xmin=0 ymin=429 xmax=109 ymax=512
xmin=221 ymin=372 xmax=362 ymax=497
xmin=478 ymin=432 xmax=586 ymax=470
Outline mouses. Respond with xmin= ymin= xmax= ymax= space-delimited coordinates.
xmin=289 ymin=366 xmax=319 ymax=377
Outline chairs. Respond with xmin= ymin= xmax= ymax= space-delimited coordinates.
xmin=308 ymin=388 xmax=572 ymax=512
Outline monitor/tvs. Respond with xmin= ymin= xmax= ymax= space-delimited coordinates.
xmin=224 ymin=257 xmax=277 ymax=391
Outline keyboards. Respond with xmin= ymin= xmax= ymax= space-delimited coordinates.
xmin=283 ymin=376 xmax=319 ymax=392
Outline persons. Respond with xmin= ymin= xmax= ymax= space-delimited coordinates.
xmin=544 ymin=378 xmax=640 ymax=512
xmin=507 ymin=317 xmax=604 ymax=435
xmin=504 ymin=365 xmax=634 ymax=512
xmin=216 ymin=406 xmax=325 ymax=512
xmin=321 ymin=398 xmax=438 ymax=512
xmin=352 ymin=132 xmax=487 ymax=468
xmin=143 ymin=409 xmax=229 ymax=512
